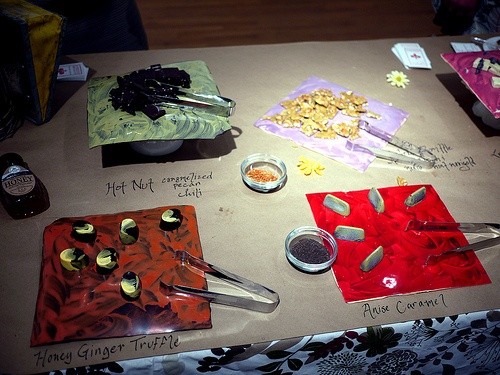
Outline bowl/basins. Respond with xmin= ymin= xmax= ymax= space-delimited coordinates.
xmin=133 ymin=141 xmax=182 ymax=155
xmin=240 ymin=153 xmax=287 ymax=192
xmin=285 ymin=226 xmax=338 ymax=272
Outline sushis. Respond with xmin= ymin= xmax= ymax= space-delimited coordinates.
xmin=120 ymin=271 xmax=142 ymax=301
xmin=72 ymin=220 xmax=96 ymax=243
xmin=96 ymin=247 xmax=118 ymax=275
xmin=59 ymin=248 xmax=89 ymax=272
xmin=119 ymin=218 xmax=138 ymax=245
xmin=160 ymin=209 xmax=182 ymax=230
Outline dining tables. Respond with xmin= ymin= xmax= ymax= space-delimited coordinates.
xmin=0 ymin=35 xmax=500 ymax=375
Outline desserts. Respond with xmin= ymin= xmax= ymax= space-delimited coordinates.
xmin=359 ymin=245 xmax=383 ymax=271
xmin=322 ymin=194 xmax=351 ymax=217
xmin=269 ymin=87 xmax=382 ymax=140
xmin=334 ymin=225 xmax=364 ymax=241
xmin=404 ymin=186 xmax=427 ymax=207
xmin=472 ymin=57 xmax=499 ymax=89
xmin=368 ymin=187 xmax=385 ymax=213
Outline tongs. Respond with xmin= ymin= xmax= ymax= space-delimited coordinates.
xmin=160 ymin=251 xmax=279 ymax=314
xmin=137 ymin=78 xmax=236 ymax=117
xmin=404 ymin=219 xmax=500 ymax=265
xmin=346 ymin=120 xmax=437 ymax=169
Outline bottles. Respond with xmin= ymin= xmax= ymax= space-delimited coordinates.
xmin=1 ymin=152 xmax=49 ymax=220
